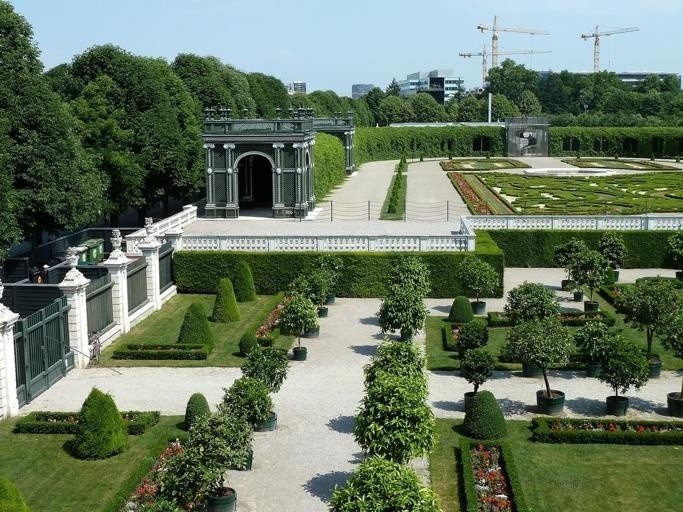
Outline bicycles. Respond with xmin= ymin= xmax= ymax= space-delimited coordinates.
xmin=88 ymin=330 xmax=103 ymax=364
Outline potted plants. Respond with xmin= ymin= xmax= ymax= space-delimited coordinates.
xmin=301 ymin=254 xmax=341 ymax=319
xmin=334 ymin=257 xmax=441 ymax=512
xmin=504 ymin=318 xmax=572 ymax=416
xmin=666 ymin=231 xmax=683 ymax=280
xmin=660 ymin=311 xmax=683 ymax=416
xmin=602 ymin=335 xmax=649 ymax=415
xmin=222 ymin=376 xmax=275 ymax=473
xmin=241 ymin=343 xmax=292 ymax=433
xmin=504 ymin=281 xmax=566 ymax=378
xmin=126 ymin=405 xmax=255 ymax=512
xmin=630 ymin=277 xmax=683 ymax=377
xmin=553 ymin=231 xmax=629 ymax=313
xmin=574 ymin=321 xmax=613 ymax=377
xmin=460 ymin=349 xmax=495 ymax=410
xmin=455 ymin=256 xmax=500 ymax=314
xmin=278 ymin=288 xmax=320 ymax=361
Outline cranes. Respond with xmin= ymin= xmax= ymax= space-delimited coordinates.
xmin=476 ymin=16 xmax=553 ymax=68
xmin=575 ymin=22 xmax=640 ymax=83
xmin=457 ymin=44 xmax=552 ymax=88
